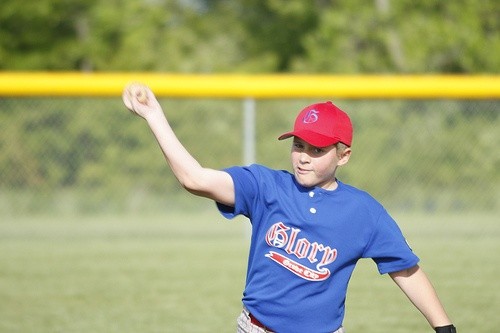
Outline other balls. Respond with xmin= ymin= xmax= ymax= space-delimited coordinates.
xmin=127 ymin=89 xmax=148 ymax=103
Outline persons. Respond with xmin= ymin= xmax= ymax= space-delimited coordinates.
xmin=122 ymin=81 xmax=458 ymax=333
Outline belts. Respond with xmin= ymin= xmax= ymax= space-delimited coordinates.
xmin=249 ymin=313 xmax=273 ymax=332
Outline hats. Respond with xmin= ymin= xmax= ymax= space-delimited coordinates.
xmin=278 ymin=101 xmax=353 ymax=148
